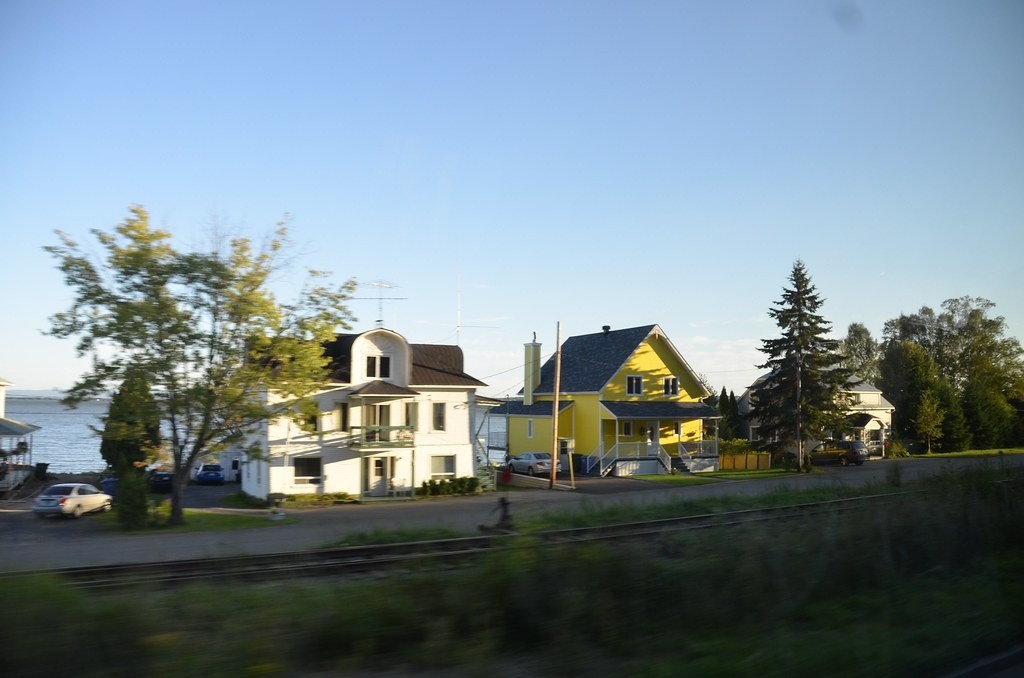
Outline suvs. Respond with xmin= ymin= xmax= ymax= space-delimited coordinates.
xmin=148 ymin=473 xmax=175 ymax=493
xmin=194 ymin=464 xmax=226 ymax=486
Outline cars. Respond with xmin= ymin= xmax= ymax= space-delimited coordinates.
xmin=507 ymin=453 xmax=561 ymax=476
xmin=99 ymin=479 xmax=120 ymax=497
xmin=32 ymin=483 xmax=113 ymax=519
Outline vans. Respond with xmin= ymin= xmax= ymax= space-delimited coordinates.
xmin=809 ymin=441 xmax=869 ymax=466
